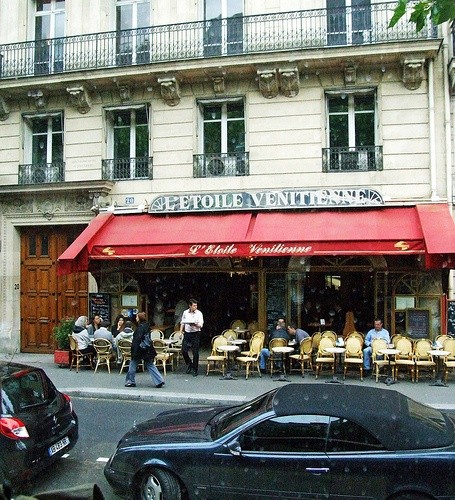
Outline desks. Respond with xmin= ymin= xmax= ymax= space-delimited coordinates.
xmin=335 ymin=340 xmax=347 ymax=366
xmin=217 ymin=345 xmax=240 ymax=380
xmin=230 ymin=338 xmax=248 ymax=369
xmin=160 ymin=339 xmax=177 ymax=369
xmin=426 ymin=350 xmax=452 ymax=387
xmin=323 ymin=347 xmax=344 ymax=384
xmin=377 ymin=347 xmax=401 ymax=386
xmin=239 ymin=328 xmax=250 ymax=340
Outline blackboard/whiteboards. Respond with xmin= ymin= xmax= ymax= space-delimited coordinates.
xmin=405 ymin=307 xmax=433 ymax=341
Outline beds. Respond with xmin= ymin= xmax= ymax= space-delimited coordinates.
xmin=266 ymin=337 xmax=288 ymax=377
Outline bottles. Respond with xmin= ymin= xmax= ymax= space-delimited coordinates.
xmin=252 ymin=323 xmax=255 ymax=329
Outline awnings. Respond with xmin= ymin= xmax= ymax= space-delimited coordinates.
xmin=55 ymin=204 xmax=455 ymax=276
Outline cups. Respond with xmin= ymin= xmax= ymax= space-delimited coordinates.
xmin=436 ymin=347 xmax=439 ymax=351
xmin=236 ymin=327 xmax=239 ymax=330
xmin=293 ymin=339 xmax=295 ymax=342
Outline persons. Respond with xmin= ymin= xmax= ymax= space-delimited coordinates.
xmin=72 ymin=314 xmax=134 ymax=365
xmin=153 ymin=293 xmax=188 ymax=331
xmin=260 ymin=299 xmax=364 ymax=374
xmin=179 ymin=299 xmax=204 ymax=377
xmin=123 ymin=311 xmax=165 ymax=388
xmin=362 ymin=318 xmax=391 ymax=377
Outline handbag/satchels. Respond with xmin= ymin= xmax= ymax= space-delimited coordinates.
xmin=140 ymin=323 xmax=154 ymax=350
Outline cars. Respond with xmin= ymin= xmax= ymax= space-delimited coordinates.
xmin=0 ymin=361 xmax=79 ymax=486
xmin=104 ymin=383 xmax=455 ymax=500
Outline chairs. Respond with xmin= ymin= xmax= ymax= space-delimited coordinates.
xmin=221 ymin=328 xmax=239 ymax=366
xmin=271 ymin=346 xmax=295 ymax=382
xmin=288 ymin=336 xmax=312 ymax=377
xmin=248 ymin=320 xmax=259 ymax=336
xmin=234 ymin=334 xmax=262 ymax=380
xmin=67 ymin=333 xmax=95 ymax=374
xmin=312 ymin=328 xmax=455 ymax=386
xmin=206 ymin=333 xmax=229 ymax=377
xmin=117 ymin=338 xmax=144 ymax=376
xmin=168 ymin=330 xmax=185 ymax=367
xmin=230 ymin=319 xmax=246 ymax=329
xmin=241 ymin=327 xmax=266 ymax=373
xmin=149 ymin=328 xmax=166 ymax=340
xmin=149 ymin=338 xmax=174 ymax=375
xmin=91 ymin=337 xmax=117 ymax=373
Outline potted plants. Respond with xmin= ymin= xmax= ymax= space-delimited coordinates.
xmin=53 ymin=314 xmax=78 ymax=365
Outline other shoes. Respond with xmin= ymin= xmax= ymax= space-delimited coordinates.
xmin=186 ymin=363 xmax=193 ymax=374
xmin=125 ymin=382 xmax=136 ymax=387
xmin=362 ymin=370 xmax=370 ymax=377
xmin=192 ymin=371 xmax=198 ymax=377
xmin=260 ymin=369 xmax=266 ymax=374
xmin=153 ymin=382 xmax=165 ymax=388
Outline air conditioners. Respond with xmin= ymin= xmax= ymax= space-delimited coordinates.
xmin=339 ymin=150 xmax=368 ymax=171
xmin=202 ymin=156 xmax=237 ymax=177
xmin=30 ymin=167 xmax=59 ymax=182
